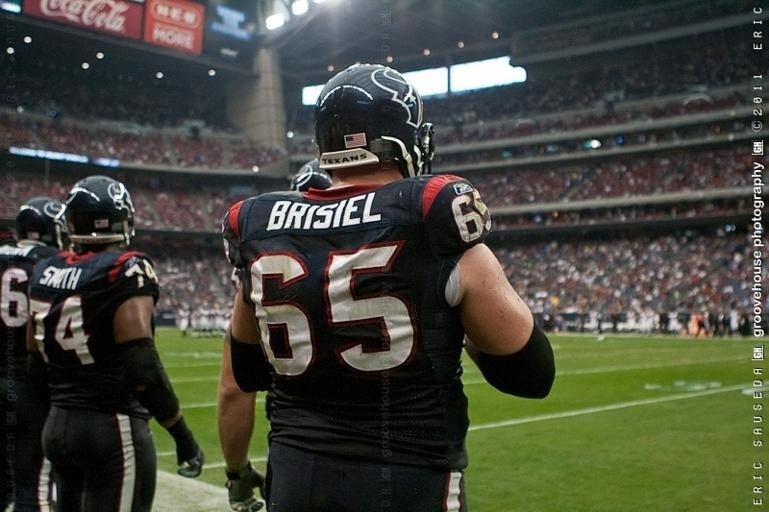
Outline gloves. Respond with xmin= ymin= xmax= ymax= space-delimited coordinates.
xmin=168 ymin=417 xmax=205 ymax=478
xmin=227 ymin=460 xmax=267 ymax=511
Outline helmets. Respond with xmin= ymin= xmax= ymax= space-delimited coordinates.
xmin=15 ymin=176 xmax=135 ymax=248
xmin=314 ymin=63 xmax=435 ymax=178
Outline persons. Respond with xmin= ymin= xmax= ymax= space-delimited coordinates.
xmin=218 ymin=60 xmax=555 ymax=510
xmin=0 ymin=194 xmax=72 ymax=512
xmin=23 ymin=173 xmax=206 ymax=511
xmin=0 ymin=1 xmax=769 ymax=343
xmin=288 ymin=155 xmax=333 ymax=194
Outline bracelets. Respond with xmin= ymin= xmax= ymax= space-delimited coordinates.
xmin=223 ymin=462 xmax=253 ymax=479
